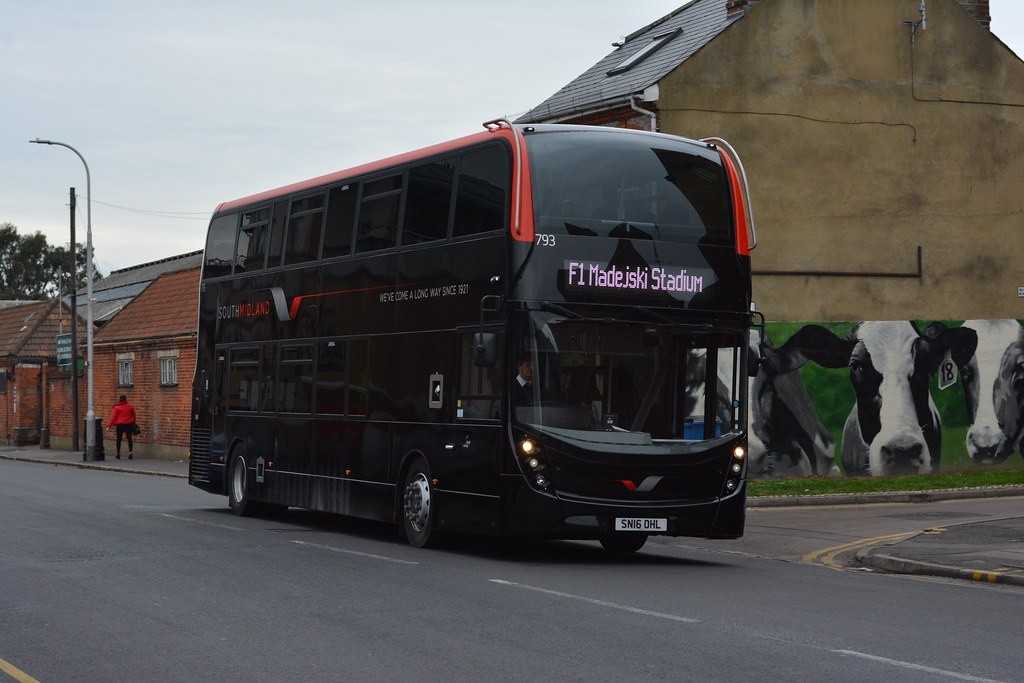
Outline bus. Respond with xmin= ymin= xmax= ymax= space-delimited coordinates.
xmin=187 ymin=117 xmax=765 ymax=555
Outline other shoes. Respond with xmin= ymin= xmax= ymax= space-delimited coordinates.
xmin=115 ymin=453 xmax=120 ymax=459
xmin=128 ymin=453 xmax=133 ymax=460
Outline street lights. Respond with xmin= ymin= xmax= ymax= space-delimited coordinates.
xmin=29 ymin=139 xmax=96 ymax=461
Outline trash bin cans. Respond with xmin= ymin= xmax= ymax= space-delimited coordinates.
xmin=83 ymin=417 xmax=106 ymax=462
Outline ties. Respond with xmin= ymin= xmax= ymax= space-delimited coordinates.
xmin=523 ymin=383 xmax=529 ymax=392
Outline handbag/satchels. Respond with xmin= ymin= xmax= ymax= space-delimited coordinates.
xmin=133 ymin=424 xmax=140 ymax=435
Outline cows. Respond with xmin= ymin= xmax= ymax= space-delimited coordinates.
xmin=684 ymin=319 xmax=1023 ymax=476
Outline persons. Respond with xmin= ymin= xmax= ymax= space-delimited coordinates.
xmin=106 ymin=394 xmax=141 ymax=461
xmin=511 ymin=350 xmax=552 ymax=407
